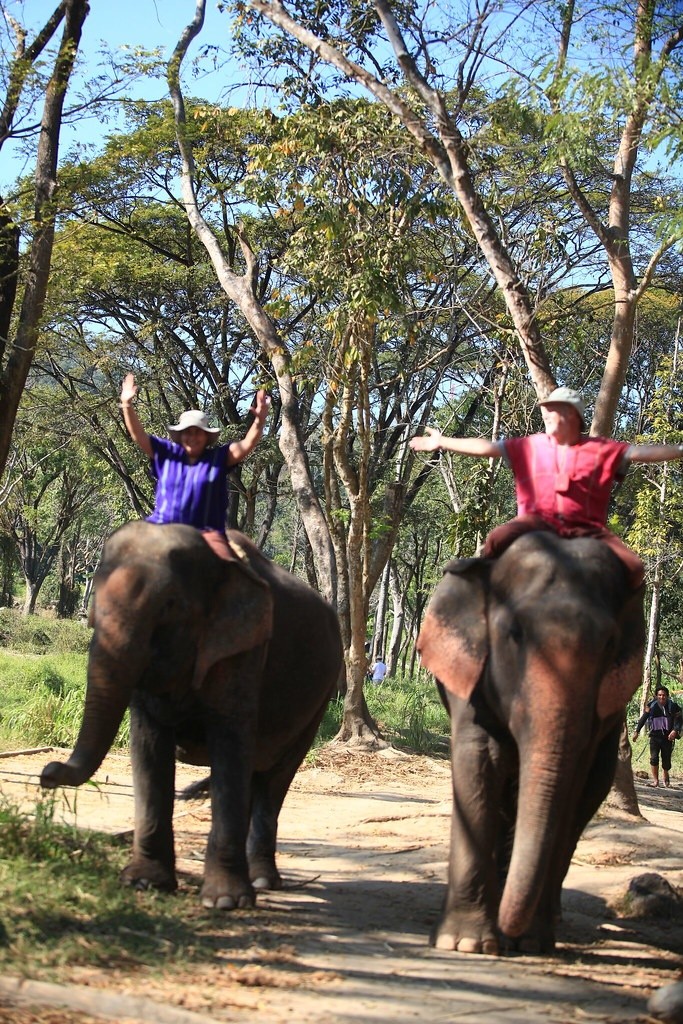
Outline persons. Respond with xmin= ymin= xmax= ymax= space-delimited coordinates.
xmin=632 ymin=686 xmax=682 ymax=787
xmin=369 ymin=655 xmax=386 ymax=688
xmin=409 ymin=388 xmax=683 ymax=591
xmin=121 ymin=372 xmax=270 ymax=588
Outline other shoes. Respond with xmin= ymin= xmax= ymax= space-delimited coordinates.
xmin=662 ymin=773 xmax=669 ymax=788
xmin=653 ymin=779 xmax=660 ymax=787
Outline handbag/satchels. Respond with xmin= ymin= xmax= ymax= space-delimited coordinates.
xmin=669 ymin=699 xmax=683 ymax=740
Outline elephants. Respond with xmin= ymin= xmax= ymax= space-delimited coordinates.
xmin=39 ymin=519 xmax=344 ymax=911
xmin=416 ymin=517 xmax=646 ymax=955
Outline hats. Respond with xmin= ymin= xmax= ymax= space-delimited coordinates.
xmin=167 ymin=409 xmax=222 ymax=447
xmin=537 ymin=387 xmax=587 ymax=433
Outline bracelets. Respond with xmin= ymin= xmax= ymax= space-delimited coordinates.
xmin=678 ymin=445 xmax=683 ymax=458
